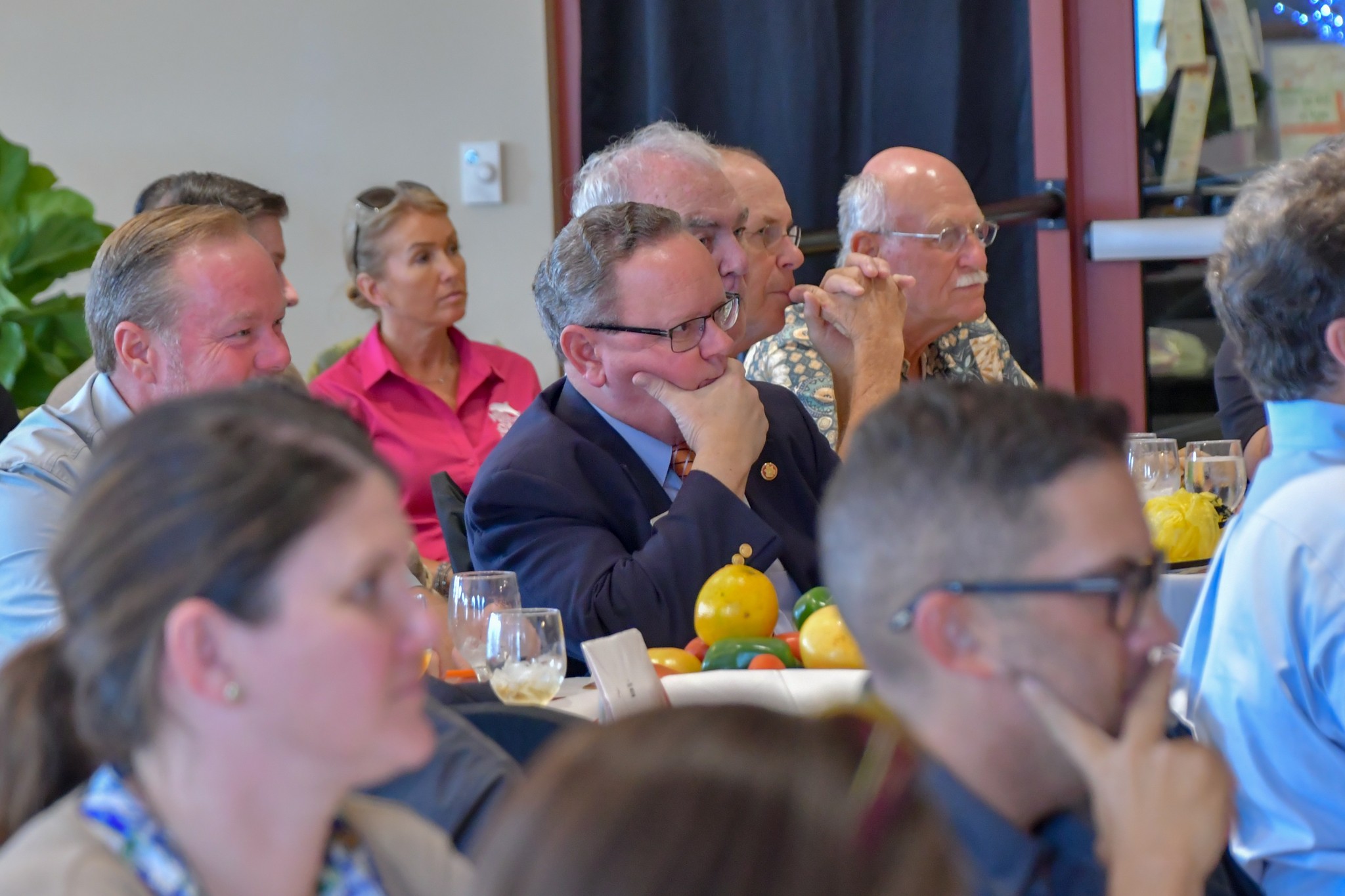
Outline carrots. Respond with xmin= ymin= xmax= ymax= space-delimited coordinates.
xmin=773 ymin=631 xmax=803 ymax=663
xmin=684 ymin=637 xmax=711 ymax=663
xmin=747 ymin=654 xmax=786 ymax=670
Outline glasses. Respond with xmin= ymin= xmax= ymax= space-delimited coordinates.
xmin=879 ymin=221 xmax=999 ymax=252
xmin=744 ymin=223 xmax=801 ymax=253
xmin=579 ymin=292 xmax=740 ymax=353
xmin=352 ymin=181 xmax=433 ymax=267
xmin=889 ymin=548 xmax=1165 ymax=632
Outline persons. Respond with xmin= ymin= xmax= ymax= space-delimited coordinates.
xmin=1 ymin=123 xmax=1266 ymax=663
xmin=0 ymin=377 xmax=473 ymax=896
xmin=461 ymin=706 xmax=972 ymax=896
xmin=1171 ymin=139 xmax=1345 ymax=896
xmin=461 ymin=202 xmax=843 ymax=680
xmin=815 ymin=377 xmax=1273 ymax=896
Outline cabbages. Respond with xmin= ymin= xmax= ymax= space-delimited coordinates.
xmin=1138 ymin=486 xmax=1222 ymax=565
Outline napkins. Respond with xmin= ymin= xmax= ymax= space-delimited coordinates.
xmin=660 ymin=668 xmax=873 ymax=715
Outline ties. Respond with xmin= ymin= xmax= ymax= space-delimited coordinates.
xmin=671 ymin=441 xmax=696 ymax=478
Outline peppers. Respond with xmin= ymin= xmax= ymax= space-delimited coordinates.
xmin=701 ymin=636 xmax=799 ymax=673
xmin=792 ymin=585 xmax=836 ymax=628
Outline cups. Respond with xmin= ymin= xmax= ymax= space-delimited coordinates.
xmin=1128 ymin=439 xmax=1181 ymax=509
xmin=448 ymin=570 xmax=522 ymax=683
xmin=1122 ymin=433 xmax=1157 ymax=453
xmin=485 ymin=607 xmax=567 ymax=707
xmin=1184 ymin=439 xmax=1245 ymax=528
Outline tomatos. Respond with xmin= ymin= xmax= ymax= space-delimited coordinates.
xmin=648 ymin=564 xmax=866 ymax=675
